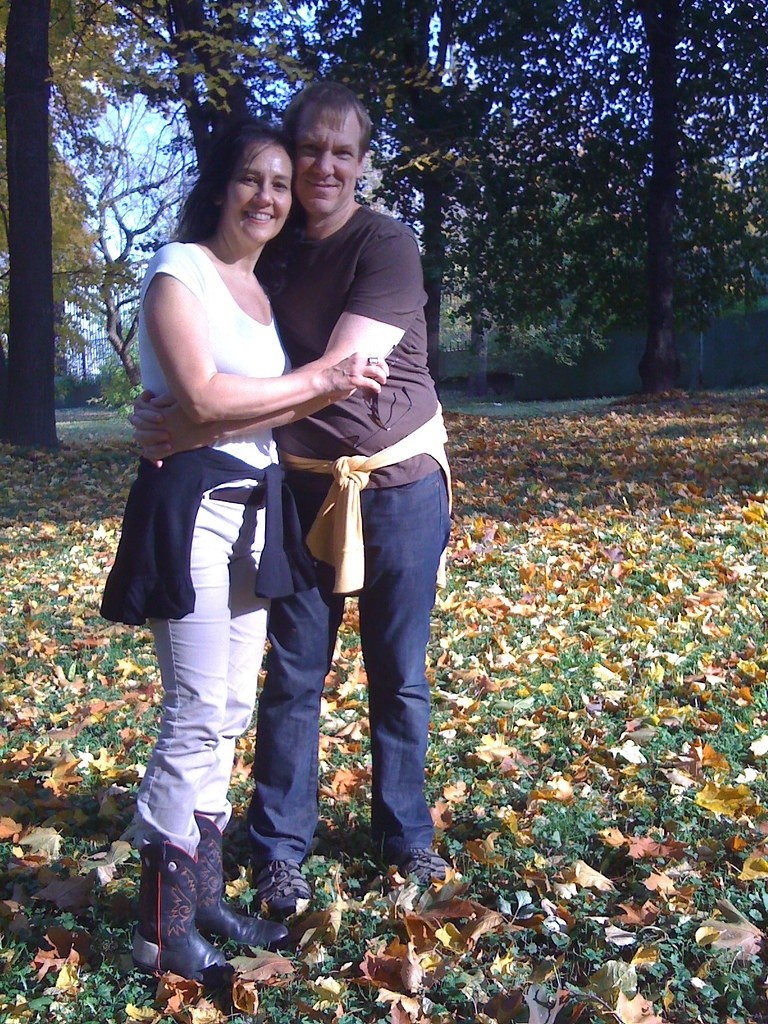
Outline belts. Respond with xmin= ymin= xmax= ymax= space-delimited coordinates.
xmin=203 ymin=486 xmax=267 ymax=509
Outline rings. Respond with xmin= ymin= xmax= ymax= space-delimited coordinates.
xmin=368 ymin=356 xmax=379 ymax=364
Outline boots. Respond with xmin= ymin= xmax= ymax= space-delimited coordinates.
xmin=133 ymin=841 xmax=228 ymax=981
xmin=194 ymin=813 xmax=289 ymax=949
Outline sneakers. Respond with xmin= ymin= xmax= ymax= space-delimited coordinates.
xmin=252 ymin=858 xmax=314 ymax=917
xmin=390 ymin=846 xmax=452 ymax=887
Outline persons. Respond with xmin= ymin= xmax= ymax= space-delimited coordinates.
xmin=130 ymin=81 xmax=455 ymax=987
